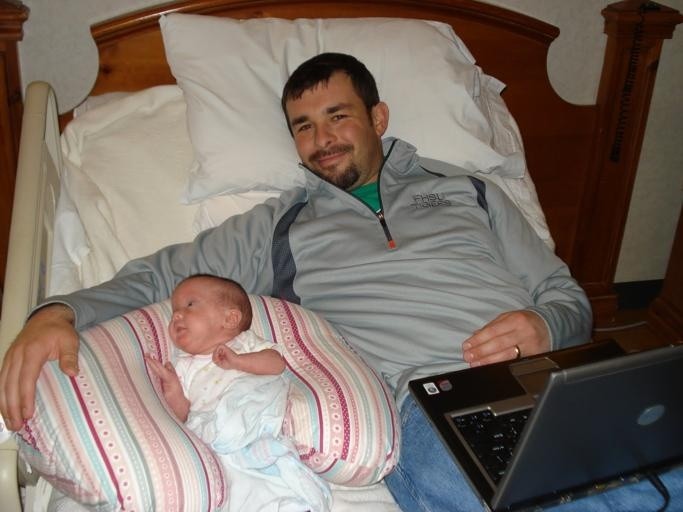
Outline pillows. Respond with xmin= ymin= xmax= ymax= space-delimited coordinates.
xmin=14 ymin=294 xmax=401 ymax=512
xmin=157 ymin=10 xmax=525 ymax=207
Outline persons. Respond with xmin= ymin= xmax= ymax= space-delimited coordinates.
xmin=143 ymin=273 xmax=289 ymax=424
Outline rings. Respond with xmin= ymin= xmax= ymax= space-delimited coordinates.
xmin=514 ymin=344 xmax=521 ymax=359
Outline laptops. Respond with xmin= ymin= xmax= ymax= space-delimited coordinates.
xmin=409 ymin=336 xmax=682 ymax=512
